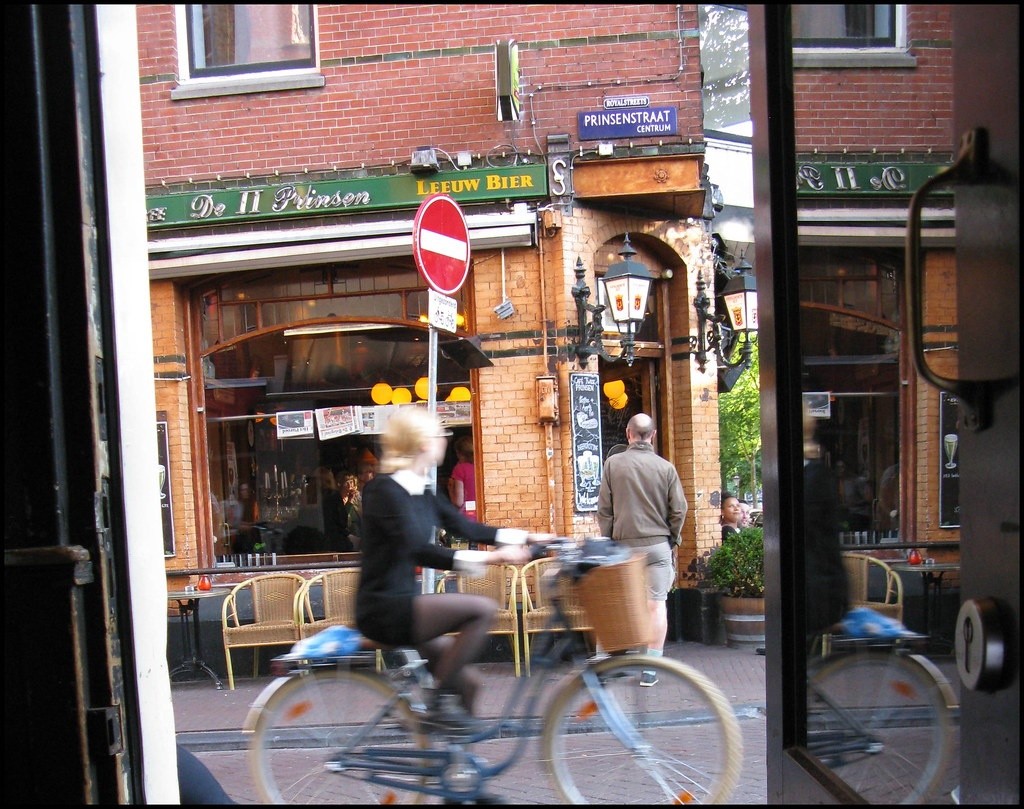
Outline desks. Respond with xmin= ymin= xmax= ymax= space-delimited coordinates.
xmin=167 ymin=586 xmax=231 ymax=690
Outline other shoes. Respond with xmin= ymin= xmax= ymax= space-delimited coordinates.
xmin=444 ymin=790 xmax=504 ymax=805
xmin=415 ymin=699 xmax=493 ymax=735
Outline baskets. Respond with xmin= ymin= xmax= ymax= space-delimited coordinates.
xmin=576 ymin=549 xmax=654 ymax=651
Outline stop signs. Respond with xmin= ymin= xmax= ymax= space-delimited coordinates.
xmin=413 ymin=192 xmax=472 ymax=295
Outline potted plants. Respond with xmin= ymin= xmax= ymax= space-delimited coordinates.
xmin=704 ymin=525 xmax=764 ymax=650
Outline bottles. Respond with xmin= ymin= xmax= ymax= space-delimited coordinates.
xmin=909 ymin=549 xmax=922 ymax=565
xmin=199 ymin=575 xmax=211 ymax=591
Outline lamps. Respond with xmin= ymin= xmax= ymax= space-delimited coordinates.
xmin=688 ymin=249 xmax=759 ymax=374
xmin=603 ymin=379 xmax=628 ymax=409
xmin=573 ymin=232 xmax=654 ymax=368
xmin=370 ymin=372 xmax=471 ymax=404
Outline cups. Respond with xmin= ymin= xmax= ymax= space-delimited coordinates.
xmin=185 ymin=585 xmax=194 ymax=595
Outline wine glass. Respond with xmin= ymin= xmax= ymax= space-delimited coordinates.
xmin=944 ymin=435 xmax=958 ymax=469
xmin=159 ymin=464 xmax=166 ymax=499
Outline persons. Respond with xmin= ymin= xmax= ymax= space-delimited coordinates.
xmin=445 ymin=435 xmax=477 ymax=527
xmin=595 ymin=413 xmax=688 ymax=690
xmin=721 ymin=492 xmax=744 ymax=543
xmin=352 ymin=406 xmax=552 ymax=735
xmin=307 ymin=468 xmax=357 ymax=554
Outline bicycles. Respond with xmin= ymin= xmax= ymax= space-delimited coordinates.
xmin=802 ymin=607 xmax=958 ymax=805
xmin=239 ymin=531 xmax=744 ymax=805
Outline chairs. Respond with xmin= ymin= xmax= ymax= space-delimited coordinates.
xmin=434 ymin=563 xmax=521 ymax=677
xmin=297 ymin=567 xmax=387 ymax=673
xmin=520 ymin=556 xmax=603 ymax=677
xmin=221 ymin=573 xmax=314 ymax=690
xmin=817 ymin=548 xmax=904 ymax=663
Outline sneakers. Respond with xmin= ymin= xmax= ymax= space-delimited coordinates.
xmin=640 ymin=671 xmax=659 ymax=687
xmin=598 ymin=677 xmax=606 ymax=684
